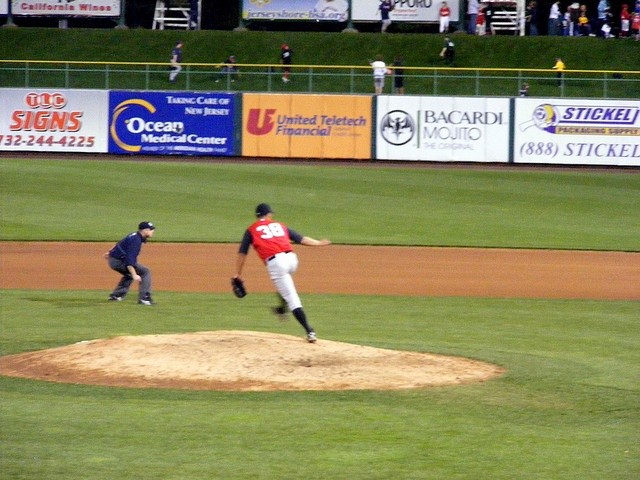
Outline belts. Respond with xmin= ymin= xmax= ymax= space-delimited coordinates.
xmin=269 ymin=251 xmax=291 ymax=260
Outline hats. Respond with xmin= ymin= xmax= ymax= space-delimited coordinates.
xmin=256 ymin=203 xmax=274 ymax=215
xmin=139 ymin=221 xmax=155 ymax=229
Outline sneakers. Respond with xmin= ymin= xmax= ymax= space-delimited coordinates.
xmin=108 ymin=294 xmax=122 ymax=301
xmin=306 ymin=332 xmax=316 ymax=340
xmin=138 ymin=298 xmax=156 ymax=304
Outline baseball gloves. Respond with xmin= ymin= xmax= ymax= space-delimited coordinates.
xmin=231 ymin=277 xmax=246 ymax=298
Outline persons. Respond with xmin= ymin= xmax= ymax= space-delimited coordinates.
xmin=518 ymin=83 xmax=529 ymax=96
xmin=466 ymin=0 xmax=494 ymax=35
xmin=102 ymin=222 xmax=157 ymax=306
xmin=388 ymin=54 xmax=404 ymax=94
xmin=371 ymin=55 xmax=392 ymax=94
xmin=214 ymin=56 xmax=241 ymax=83
xmin=169 ymin=41 xmax=183 ymax=81
xmin=439 ymin=1 xmax=450 ymax=33
xmin=375 ymin=0 xmax=394 ymax=33
xmin=525 ymin=2 xmax=537 ymax=35
xmin=562 ymin=0 xmax=640 ymax=37
xmin=548 ymin=1 xmax=561 ymax=35
xmin=553 ymin=57 xmax=565 ymax=86
xmin=230 ymin=202 xmax=332 ymax=343
xmin=440 ymin=38 xmax=455 ymax=64
xmin=278 ymin=44 xmax=293 ymax=83
